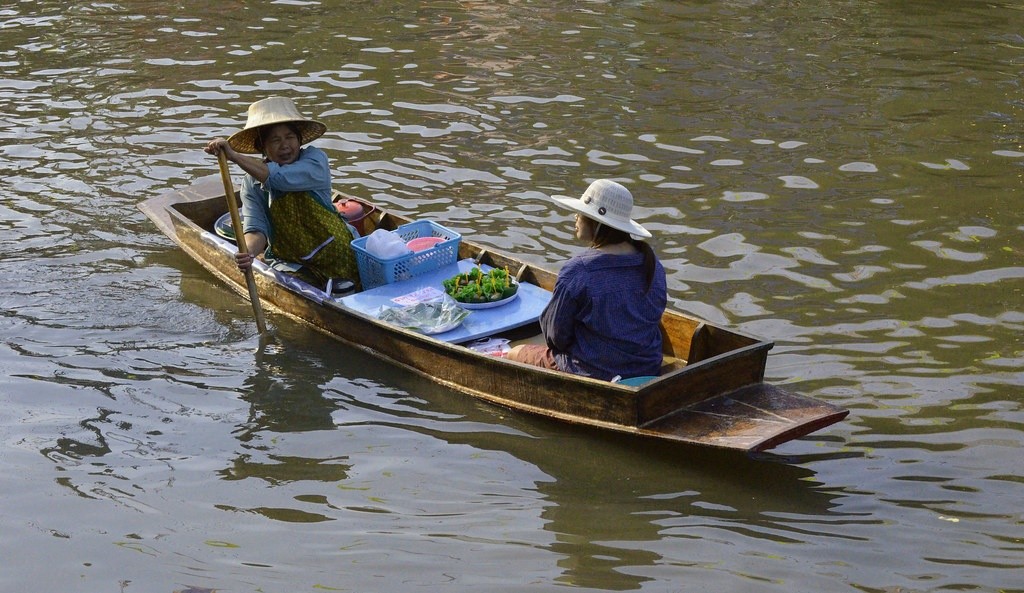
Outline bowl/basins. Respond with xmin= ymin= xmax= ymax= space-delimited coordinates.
xmin=214 ymin=207 xmax=242 ymax=246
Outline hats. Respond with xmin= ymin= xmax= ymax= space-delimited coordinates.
xmin=225 ymin=97 xmax=328 ymax=154
xmin=551 ymin=178 xmax=653 ymax=239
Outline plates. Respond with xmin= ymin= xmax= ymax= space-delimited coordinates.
xmin=443 ymin=276 xmax=521 ymax=309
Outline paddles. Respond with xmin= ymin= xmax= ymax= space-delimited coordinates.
xmin=218 ymin=146 xmax=277 ymax=343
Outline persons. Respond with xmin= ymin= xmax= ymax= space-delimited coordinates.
xmin=505 ymin=179 xmax=668 ymax=383
xmin=204 ymin=97 xmax=361 ymax=292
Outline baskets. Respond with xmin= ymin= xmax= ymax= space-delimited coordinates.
xmin=350 ymin=219 xmax=462 ymax=290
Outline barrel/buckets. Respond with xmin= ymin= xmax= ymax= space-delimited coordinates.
xmin=333 ymin=197 xmax=376 ymax=237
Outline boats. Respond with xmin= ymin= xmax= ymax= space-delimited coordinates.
xmin=135 ymin=166 xmax=851 ymax=456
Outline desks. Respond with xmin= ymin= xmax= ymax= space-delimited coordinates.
xmin=331 ymin=257 xmax=555 ymax=346
